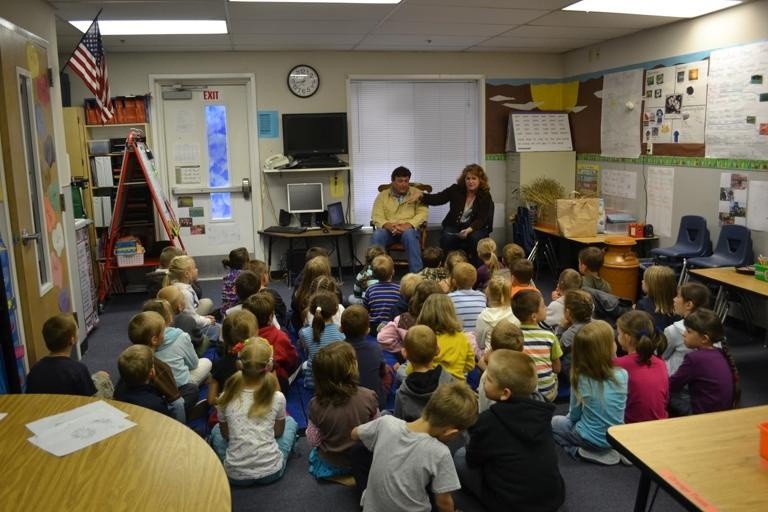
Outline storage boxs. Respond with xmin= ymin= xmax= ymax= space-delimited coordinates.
xmin=537 ymin=200 xmax=558 ymax=231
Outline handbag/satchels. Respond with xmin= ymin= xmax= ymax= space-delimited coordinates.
xmin=555 ymin=198 xmax=600 ymax=237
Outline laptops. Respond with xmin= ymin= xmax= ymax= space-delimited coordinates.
xmin=327 ymin=201 xmax=364 ymax=232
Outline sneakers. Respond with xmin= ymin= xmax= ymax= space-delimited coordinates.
xmin=325 ymin=473 xmax=356 ymax=486
xmin=190 ymin=398 xmax=209 ymax=419
xmin=577 ymin=445 xmax=621 ymax=465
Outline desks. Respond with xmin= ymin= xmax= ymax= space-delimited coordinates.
xmin=606 ymin=405 xmax=768 ymax=512
xmin=686 ymin=267 xmax=768 ymax=341
xmin=0 ymin=393 xmax=233 ymax=512
xmin=257 ymin=224 xmax=364 ymax=287
xmin=533 ymin=225 xmax=660 ymax=257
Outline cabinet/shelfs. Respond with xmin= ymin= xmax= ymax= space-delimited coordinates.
xmin=62 ymin=107 xmax=98 ymax=290
xmin=505 ymin=151 xmax=577 ymax=236
xmin=83 ymin=123 xmax=162 ymax=294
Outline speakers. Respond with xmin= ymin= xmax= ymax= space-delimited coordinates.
xmin=316 ymin=212 xmax=323 ymax=226
xmin=279 ymin=209 xmax=290 ymax=226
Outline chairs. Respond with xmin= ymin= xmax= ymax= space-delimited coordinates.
xmin=650 ymin=215 xmax=752 ymax=340
xmin=512 ymin=205 xmax=562 ymax=282
xmin=369 ymin=182 xmax=433 ymax=267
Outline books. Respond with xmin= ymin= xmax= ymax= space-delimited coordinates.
xmin=95 ymin=141 xmax=154 ymax=294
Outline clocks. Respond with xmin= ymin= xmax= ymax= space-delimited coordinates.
xmin=286 ymin=64 xmax=320 ymax=99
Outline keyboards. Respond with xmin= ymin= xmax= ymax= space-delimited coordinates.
xmin=264 ymin=225 xmax=307 ymax=234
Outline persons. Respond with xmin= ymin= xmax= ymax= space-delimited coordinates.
xmin=405 ymin=164 xmax=495 ymax=270
xmin=113 ymin=237 xmax=742 ymax=512
xmin=368 ymin=165 xmax=430 ymax=275
xmin=24 ymin=312 xmax=110 ymax=396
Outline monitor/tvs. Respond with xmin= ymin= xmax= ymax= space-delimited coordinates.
xmin=287 ymin=183 xmax=324 ymax=230
xmin=282 ymin=112 xmax=349 ymax=169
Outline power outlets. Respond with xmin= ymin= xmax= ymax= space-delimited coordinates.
xmin=646 ymin=143 xmax=653 ymax=155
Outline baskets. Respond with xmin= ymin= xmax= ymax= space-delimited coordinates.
xmin=114 ymin=248 xmax=145 ymax=267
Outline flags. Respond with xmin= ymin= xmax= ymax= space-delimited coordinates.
xmin=66 ymin=18 xmax=115 ymax=123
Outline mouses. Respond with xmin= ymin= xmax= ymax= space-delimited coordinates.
xmin=323 ymin=229 xmax=328 ymax=233
